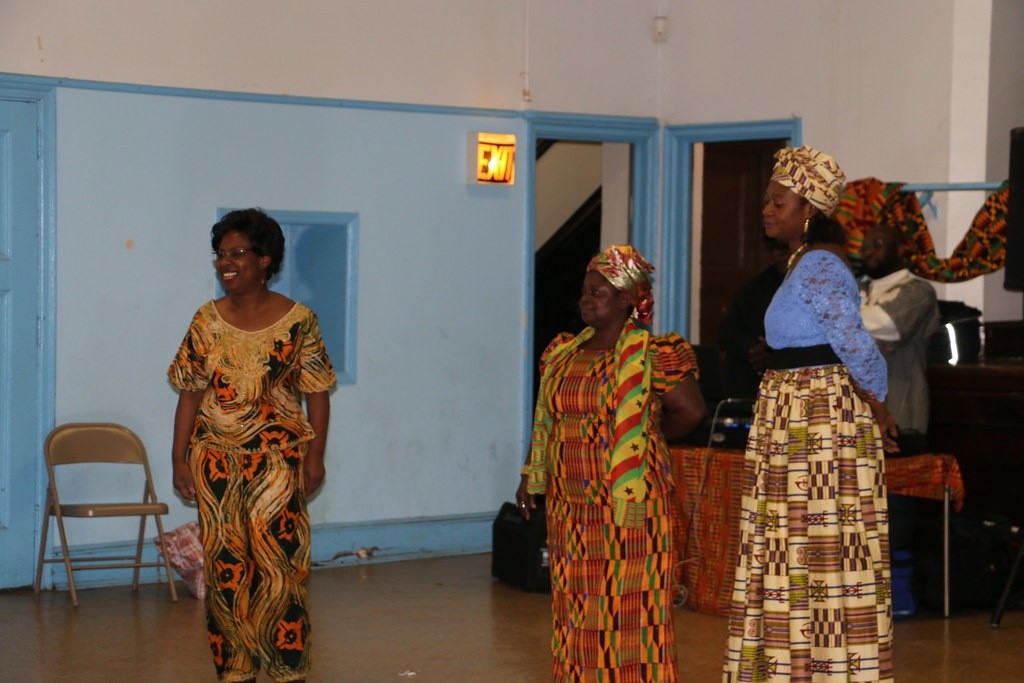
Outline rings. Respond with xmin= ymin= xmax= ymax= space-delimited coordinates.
xmin=520 ymin=500 xmax=528 ymax=511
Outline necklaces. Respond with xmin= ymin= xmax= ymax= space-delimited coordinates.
xmin=787 ymin=243 xmax=809 ymax=271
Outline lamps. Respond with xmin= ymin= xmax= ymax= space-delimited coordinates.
xmin=476 ymin=132 xmax=517 ymax=186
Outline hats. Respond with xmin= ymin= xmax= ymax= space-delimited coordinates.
xmin=772 ymin=149 xmax=843 ymax=217
xmin=585 ymin=246 xmax=655 ymax=307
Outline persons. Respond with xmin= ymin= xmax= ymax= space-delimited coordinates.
xmin=841 ymin=223 xmax=944 ymax=619
xmin=514 ymin=241 xmax=710 ymax=682
xmin=719 ymin=144 xmax=901 ymax=682
xmin=162 ymin=203 xmax=342 ymax=683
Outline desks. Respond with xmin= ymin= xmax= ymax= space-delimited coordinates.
xmin=659 ymin=446 xmax=958 ymax=621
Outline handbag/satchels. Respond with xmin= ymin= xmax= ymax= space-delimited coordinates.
xmin=153 ymin=514 xmax=212 ymax=598
xmin=489 ymin=496 xmax=550 ymax=592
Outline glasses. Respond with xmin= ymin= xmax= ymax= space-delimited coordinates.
xmin=209 ymin=244 xmax=255 ymax=263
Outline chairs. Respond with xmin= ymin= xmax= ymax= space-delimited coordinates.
xmin=33 ymin=421 xmax=179 ymax=600
